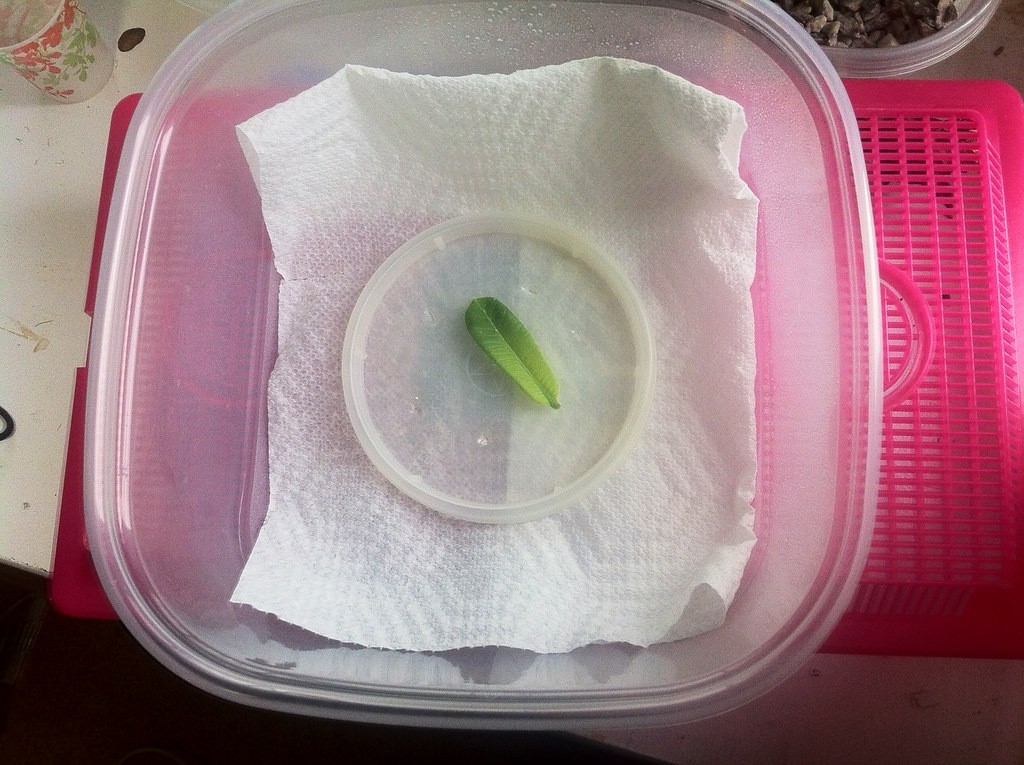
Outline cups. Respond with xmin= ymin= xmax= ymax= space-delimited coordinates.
xmin=0 ymin=0 xmax=113 ymax=104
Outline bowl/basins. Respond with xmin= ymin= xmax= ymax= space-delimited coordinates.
xmin=771 ymin=0 xmax=1001 ymax=78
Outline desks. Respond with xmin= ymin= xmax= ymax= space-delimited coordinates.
xmin=0 ymin=0 xmax=1024 ymax=765
